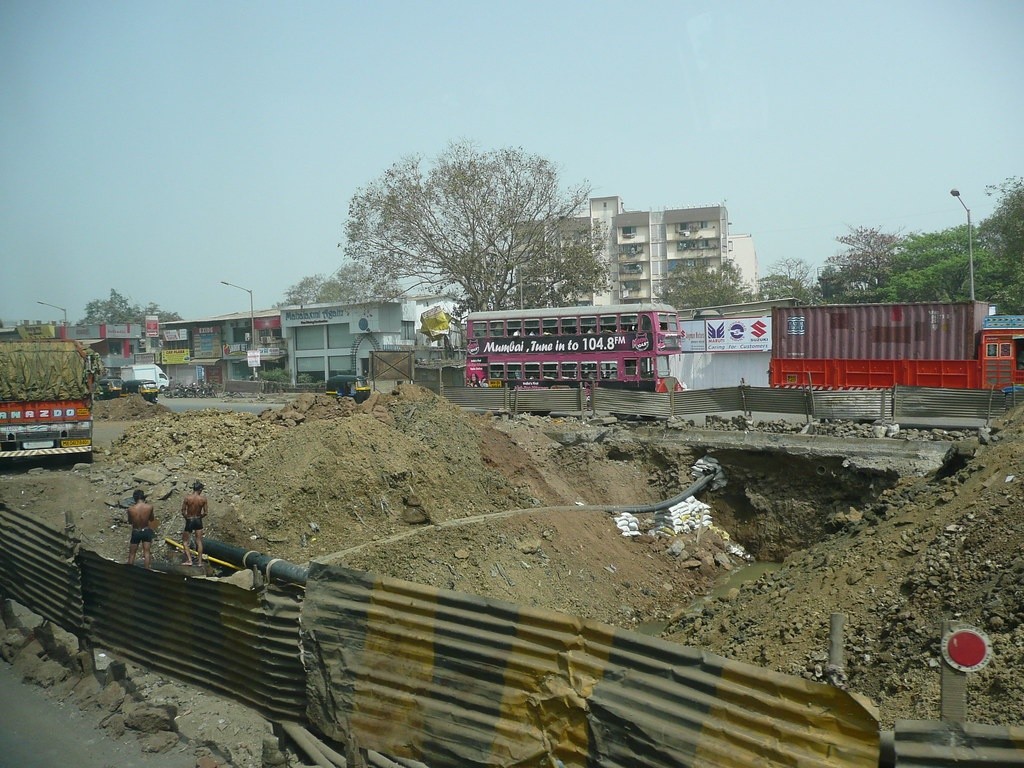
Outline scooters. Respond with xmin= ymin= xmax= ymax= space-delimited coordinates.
xmin=163 ymin=382 xmax=217 ymax=399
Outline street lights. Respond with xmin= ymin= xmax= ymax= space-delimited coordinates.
xmin=221 ymin=280 xmax=257 ymax=380
xmin=951 ymin=188 xmax=976 ymax=298
xmin=38 ymin=300 xmax=67 ymax=323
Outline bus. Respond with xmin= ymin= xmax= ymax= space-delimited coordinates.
xmin=464 ymin=303 xmax=687 ymax=421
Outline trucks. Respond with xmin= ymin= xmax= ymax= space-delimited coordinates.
xmin=0 ymin=339 xmax=96 ymax=462
xmin=121 ymin=364 xmax=173 ymax=394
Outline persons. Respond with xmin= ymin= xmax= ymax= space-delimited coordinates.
xmin=180 ymin=481 xmax=208 ymax=566
xmin=169 ymin=376 xmax=175 ymax=398
xmin=466 ymin=373 xmax=488 ymax=388
xmin=493 ymin=324 xmax=667 ymax=377
xmin=127 ymin=489 xmax=155 ymax=567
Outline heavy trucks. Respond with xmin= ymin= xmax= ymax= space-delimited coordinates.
xmin=767 ymin=301 xmax=1022 ymax=387
